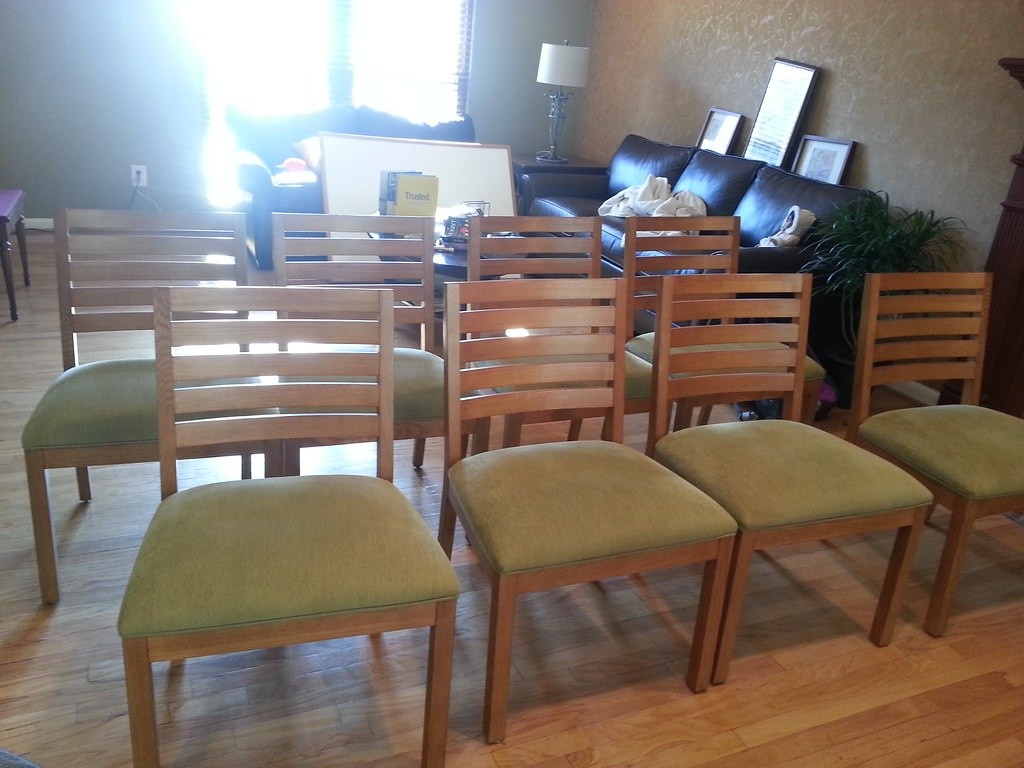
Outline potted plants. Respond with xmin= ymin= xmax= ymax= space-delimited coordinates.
xmin=798 ymin=186 xmax=980 ymax=399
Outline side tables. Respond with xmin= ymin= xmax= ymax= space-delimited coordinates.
xmin=512 ymin=152 xmax=608 ymax=201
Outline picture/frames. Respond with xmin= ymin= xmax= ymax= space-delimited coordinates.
xmin=694 ymin=107 xmax=746 ymax=155
xmin=790 ymin=135 xmax=857 ymax=185
xmin=741 ymin=57 xmax=821 ymax=169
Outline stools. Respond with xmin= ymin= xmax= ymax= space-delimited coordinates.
xmin=0 ymin=188 xmax=31 ymax=322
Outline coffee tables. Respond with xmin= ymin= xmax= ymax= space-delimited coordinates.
xmin=365 ymin=210 xmax=506 ymax=337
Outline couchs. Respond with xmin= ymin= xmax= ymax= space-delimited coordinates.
xmin=516 ymin=134 xmax=883 ymax=353
xmin=227 ymin=90 xmax=478 ymax=272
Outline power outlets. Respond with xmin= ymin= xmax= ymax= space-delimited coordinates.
xmin=129 ymin=164 xmax=147 ymax=187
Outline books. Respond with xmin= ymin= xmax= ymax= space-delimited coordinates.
xmin=441 ymin=216 xmax=487 ymax=252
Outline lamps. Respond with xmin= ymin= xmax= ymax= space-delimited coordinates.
xmin=536 ymin=39 xmax=589 ymax=164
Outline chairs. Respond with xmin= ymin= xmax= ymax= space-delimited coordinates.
xmin=22 ymin=206 xmax=1024 ymax=768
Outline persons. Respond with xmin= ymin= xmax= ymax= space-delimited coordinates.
xmin=758 ymin=205 xmax=815 ymax=247
